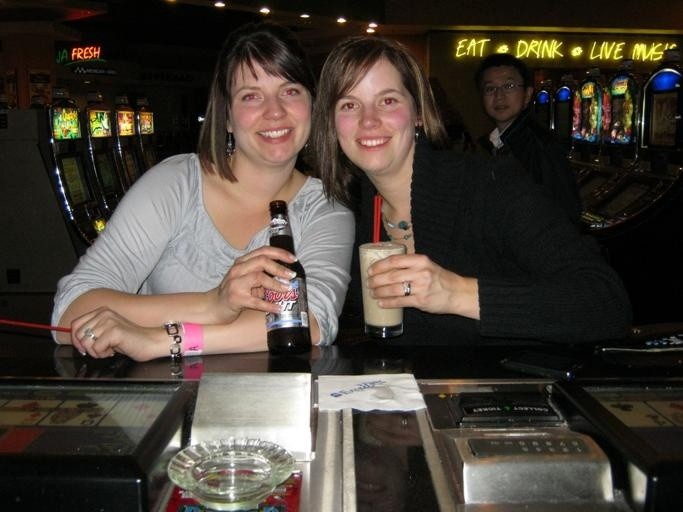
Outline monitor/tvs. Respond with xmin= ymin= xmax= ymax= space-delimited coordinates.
xmin=58 ymin=152 xmax=94 ymax=208
xmin=555 ymin=102 xmax=569 ymax=133
xmin=608 ymin=95 xmax=623 ymax=130
xmin=648 ymin=91 xmax=677 ymax=145
xmin=579 ymin=98 xmax=591 ymax=130
xmin=93 ymin=150 xmax=119 ymax=194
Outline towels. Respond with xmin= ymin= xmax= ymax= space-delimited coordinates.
xmin=318 ymin=372 xmax=428 ymax=411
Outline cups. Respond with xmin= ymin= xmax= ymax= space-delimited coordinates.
xmin=358 ymin=241 xmax=408 ymax=336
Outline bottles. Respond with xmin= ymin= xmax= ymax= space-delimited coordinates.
xmin=263 ymin=199 xmax=313 ymax=363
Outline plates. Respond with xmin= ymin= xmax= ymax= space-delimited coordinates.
xmin=166 ymin=436 xmax=297 ymax=512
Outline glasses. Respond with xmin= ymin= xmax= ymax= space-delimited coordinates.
xmin=479 ymin=81 xmax=527 ymax=96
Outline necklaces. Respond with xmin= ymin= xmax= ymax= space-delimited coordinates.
xmin=378 ymin=207 xmax=412 ymax=241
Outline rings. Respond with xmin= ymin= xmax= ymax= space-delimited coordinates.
xmin=403 ymin=282 xmax=411 ymax=296
xmin=84 ymin=329 xmax=97 ymax=341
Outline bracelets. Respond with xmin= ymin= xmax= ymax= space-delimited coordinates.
xmin=164 ymin=321 xmax=182 ymax=359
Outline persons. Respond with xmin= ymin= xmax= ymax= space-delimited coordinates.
xmin=470 ymin=54 xmax=582 ymax=223
xmin=50 ymin=21 xmax=355 ymax=363
xmin=310 ymin=37 xmax=633 ymax=342
